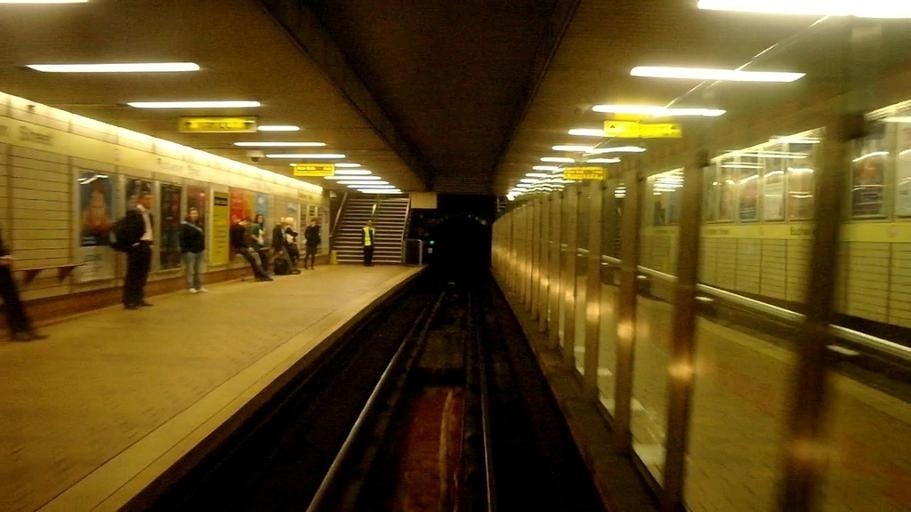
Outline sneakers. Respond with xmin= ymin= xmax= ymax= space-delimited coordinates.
xmin=127 ymin=299 xmax=153 ymax=309
xmin=13 ymin=328 xmax=49 ymax=342
xmin=189 ymin=288 xmax=207 ymax=294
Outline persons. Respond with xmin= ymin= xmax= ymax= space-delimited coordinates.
xmin=81 ymin=189 xmax=114 ymax=244
xmin=359 ymin=220 xmax=376 ymax=266
xmin=178 ymin=207 xmax=209 ymax=293
xmin=233 ymin=215 xmax=271 ymax=281
xmin=302 ymin=216 xmax=322 ymax=269
xmin=160 ymin=192 xmax=182 ymax=269
xmin=116 ymin=189 xmax=157 ymax=309
xmin=272 ymin=221 xmax=300 ymax=275
xmin=251 ymin=212 xmax=272 ymax=276
xmin=280 ymin=217 xmax=300 ymax=271
xmin=0 ymin=231 xmax=50 ymax=342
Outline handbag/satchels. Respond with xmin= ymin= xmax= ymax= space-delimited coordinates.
xmin=109 ymin=220 xmax=127 ymax=250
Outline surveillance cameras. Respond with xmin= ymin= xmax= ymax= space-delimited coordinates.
xmin=246 ymin=149 xmax=264 ymax=163
